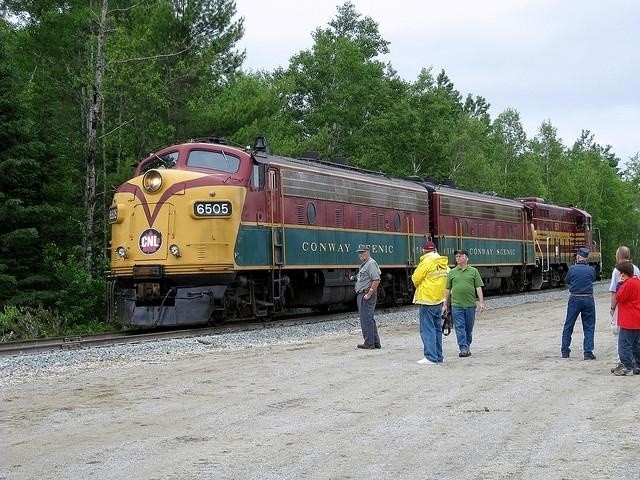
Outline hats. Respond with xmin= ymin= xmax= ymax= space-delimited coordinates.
xmin=576 ymin=248 xmax=590 ymax=258
xmin=453 ymin=249 xmax=468 ymax=256
xmin=355 ymin=244 xmax=369 ymax=252
xmin=422 ymin=241 xmax=435 ymax=250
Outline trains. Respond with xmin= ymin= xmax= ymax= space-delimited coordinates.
xmin=102 ymin=134 xmax=606 ymax=333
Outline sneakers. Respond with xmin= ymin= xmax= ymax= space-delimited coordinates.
xmin=357 ymin=343 xmax=381 ymax=349
xmin=417 ymin=358 xmax=438 ymax=365
xmin=583 ymin=351 xmax=596 ymax=360
xmin=561 ymin=351 xmax=569 ymax=358
xmin=612 ymin=363 xmax=640 ymax=376
xmin=459 ymin=349 xmax=471 ymax=357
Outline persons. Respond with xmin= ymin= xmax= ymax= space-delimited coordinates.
xmin=445 ymin=248 xmax=485 ymax=358
xmin=350 ymin=244 xmax=382 ymax=349
xmin=607 ymin=260 xmax=640 ymax=376
xmin=607 ymin=245 xmax=640 ymax=367
xmin=411 ymin=240 xmax=449 ymax=365
xmin=560 ymin=248 xmax=597 ymax=360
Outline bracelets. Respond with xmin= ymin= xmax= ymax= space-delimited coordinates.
xmin=611 ymin=306 xmax=616 ymax=311
xmin=370 ymin=288 xmax=374 ymax=291
